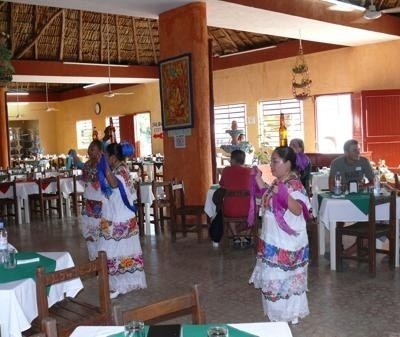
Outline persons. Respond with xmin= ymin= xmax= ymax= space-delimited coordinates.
xmin=219 ymin=150 xmax=256 ymax=249
xmin=249 ymin=147 xmax=312 ymax=325
xmin=58 ymin=125 xmax=147 ymax=299
xmin=328 ymin=139 xmax=376 ymax=258
xmin=289 ymin=139 xmax=311 ymax=188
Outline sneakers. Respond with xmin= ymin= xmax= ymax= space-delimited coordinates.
xmin=242 ymin=237 xmax=251 ymax=246
xmin=233 ymin=237 xmax=242 ymax=247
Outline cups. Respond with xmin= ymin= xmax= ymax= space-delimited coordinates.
xmin=206 ymin=325 xmax=229 ymax=337
xmin=2 ymin=248 xmax=17 ymax=270
xmin=123 ymin=318 xmax=146 ymax=337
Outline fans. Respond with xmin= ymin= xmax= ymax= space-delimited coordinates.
xmin=93 ymin=12 xmax=136 ymax=98
xmin=8 ymin=85 xmax=28 ymax=118
xmin=326 ymin=0 xmax=400 ymax=24
xmin=28 ymin=83 xmax=62 ymax=112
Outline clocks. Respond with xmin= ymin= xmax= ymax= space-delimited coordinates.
xmin=94 ymin=102 xmax=101 ymax=115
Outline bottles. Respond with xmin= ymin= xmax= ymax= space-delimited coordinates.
xmin=0 ymin=222 xmax=11 ymax=264
xmin=279 ymin=113 xmax=288 ymax=146
xmin=333 ymin=164 xmax=382 ymax=197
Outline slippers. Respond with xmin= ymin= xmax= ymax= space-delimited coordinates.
xmin=109 ymin=288 xmax=127 ymax=299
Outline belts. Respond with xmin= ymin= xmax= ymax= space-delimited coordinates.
xmin=224 ymin=190 xmax=250 ymax=196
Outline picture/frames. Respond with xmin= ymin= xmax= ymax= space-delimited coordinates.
xmin=158 ymin=52 xmax=194 ymax=131
